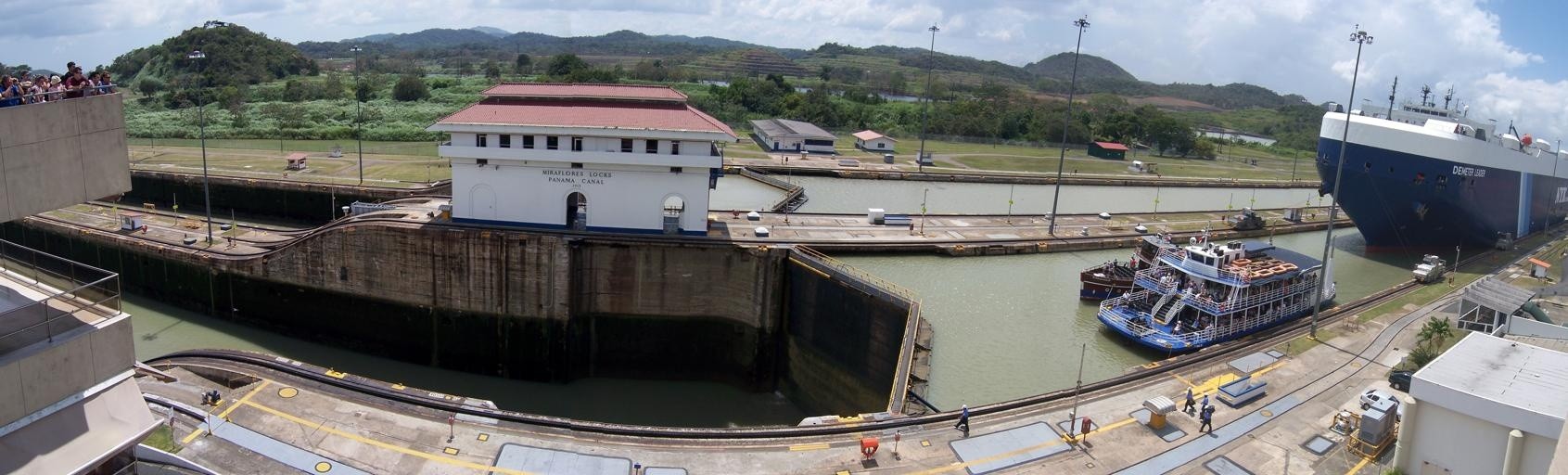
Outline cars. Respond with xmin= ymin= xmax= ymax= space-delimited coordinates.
xmin=1386 ymin=369 xmax=1416 ymax=393
xmin=1360 ymin=389 xmax=1405 ymax=423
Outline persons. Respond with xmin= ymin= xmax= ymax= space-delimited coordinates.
xmin=1200 ymin=393 xmax=1209 ymax=411
xmin=1182 ymin=386 xmax=1195 ymax=412
xmin=1104 ymin=233 xmax=1337 ymax=332
xmin=1199 ymin=409 xmax=1213 ymax=432
xmin=1 ymin=61 xmax=120 ymax=108
xmin=954 ymin=404 xmax=970 ymax=431
xmin=202 ymin=389 xmax=219 ymax=405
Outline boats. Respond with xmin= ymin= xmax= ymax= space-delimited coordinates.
xmin=1094 ymin=219 xmax=1342 ymax=356
xmin=1078 ymin=225 xmax=1192 ymax=304
xmin=1313 ymin=73 xmax=1568 ymax=255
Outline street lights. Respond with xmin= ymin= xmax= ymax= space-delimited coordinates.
xmin=187 ymin=45 xmax=218 ymax=249
xmin=1544 ymin=139 xmax=1561 ymax=236
xmin=350 ymin=41 xmax=367 ymax=186
xmin=917 ymin=20 xmax=942 ymax=180
xmin=1048 ymin=13 xmax=1090 ymax=237
xmin=1307 ymin=23 xmax=1378 ymax=342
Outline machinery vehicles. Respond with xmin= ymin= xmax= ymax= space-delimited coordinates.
xmin=1410 ymin=253 xmax=1447 ymax=286
xmin=1226 ymin=206 xmax=1268 ymax=232
xmin=1493 ymin=230 xmax=1515 ymax=252
